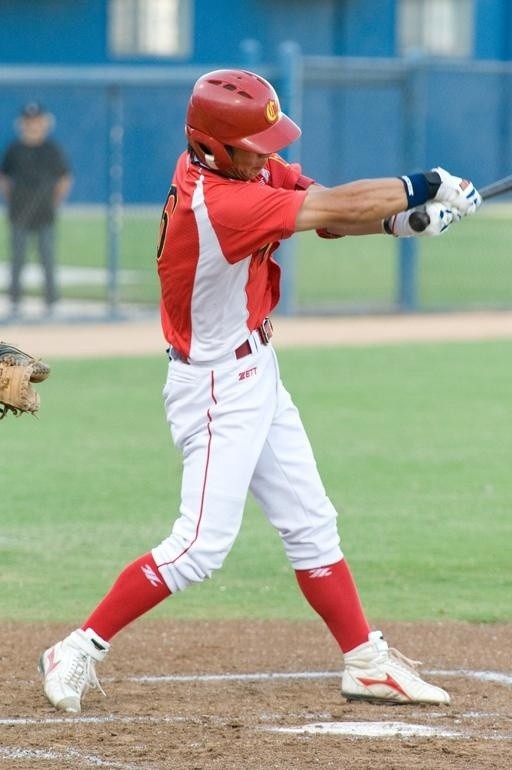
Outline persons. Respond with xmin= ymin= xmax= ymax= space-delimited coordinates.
xmin=0 ymin=95 xmax=75 ymax=320
xmin=38 ymin=65 xmax=483 ymax=717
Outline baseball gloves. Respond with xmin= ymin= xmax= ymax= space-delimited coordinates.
xmin=1 ymin=341 xmax=52 ymax=418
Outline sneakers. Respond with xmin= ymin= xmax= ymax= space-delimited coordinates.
xmin=339 ymin=631 xmax=452 ymax=705
xmin=38 ymin=628 xmax=112 ymax=717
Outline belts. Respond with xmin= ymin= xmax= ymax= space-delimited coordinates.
xmin=236 ymin=318 xmax=274 ymax=359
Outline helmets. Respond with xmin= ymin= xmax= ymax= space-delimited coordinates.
xmin=183 ymin=68 xmax=302 ymax=181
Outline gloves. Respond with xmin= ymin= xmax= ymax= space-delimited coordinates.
xmin=382 ymin=165 xmax=484 ymax=238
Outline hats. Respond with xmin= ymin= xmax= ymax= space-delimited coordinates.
xmin=17 ymin=97 xmax=49 ymax=118
